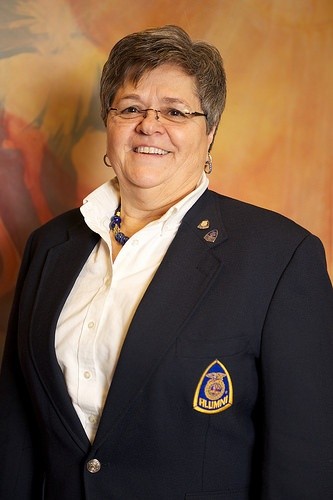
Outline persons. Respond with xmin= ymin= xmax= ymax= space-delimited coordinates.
xmin=4 ymin=25 xmax=332 ymax=499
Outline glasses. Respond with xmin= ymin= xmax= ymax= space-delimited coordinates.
xmin=105 ymin=102 xmax=208 ymax=123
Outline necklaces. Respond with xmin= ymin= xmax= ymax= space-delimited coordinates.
xmin=105 ymin=204 xmax=134 ymax=246
